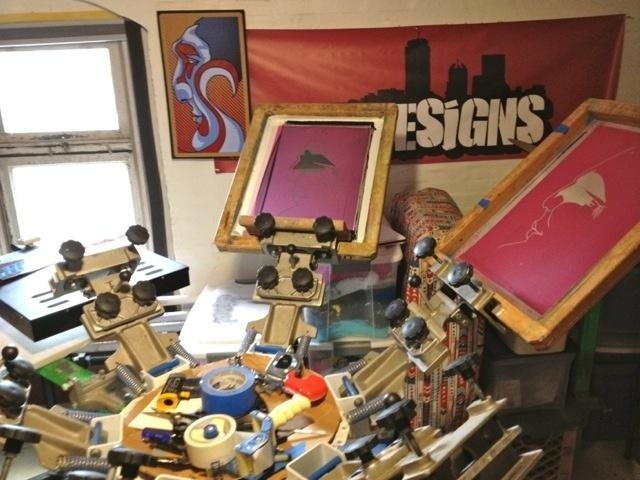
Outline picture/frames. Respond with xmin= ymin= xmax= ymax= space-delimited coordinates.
xmin=155 ymin=9 xmax=252 ymax=162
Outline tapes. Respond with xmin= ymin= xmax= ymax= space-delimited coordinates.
xmin=199 ymin=367 xmax=256 ymax=416
xmin=183 ymin=414 xmax=241 ymax=470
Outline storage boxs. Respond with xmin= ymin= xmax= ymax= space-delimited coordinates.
xmin=305 ymin=338 xmax=393 ymax=378
xmin=478 ymin=327 xmax=578 ymax=414
xmin=301 ymin=239 xmax=407 ymax=339
